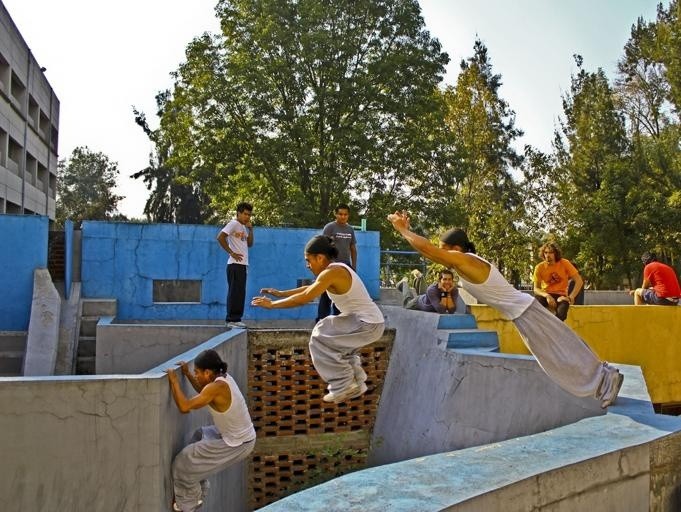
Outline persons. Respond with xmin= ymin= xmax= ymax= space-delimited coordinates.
xmin=217 ymin=202 xmax=386 ymax=405
xmin=533 ymin=241 xmax=584 ymax=322
xmin=634 ymin=251 xmax=681 ymax=306
xmin=396 ymin=269 xmax=458 ymax=314
xmin=385 ymin=210 xmax=625 ymax=410
xmin=163 ymin=349 xmax=256 ymax=512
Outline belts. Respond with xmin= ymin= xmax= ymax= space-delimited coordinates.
xmin=664 ymin=297 xmax=679 ymax=302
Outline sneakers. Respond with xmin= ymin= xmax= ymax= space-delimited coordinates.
xmin=594 ymin=361 xmax=624 ymax=409
xmin=173 ymin=499 xmax=204 ymax=511
xmin=225 ymin=321 xmax=247 ymax=329
xmin=411 ymin=269 xmax=422 ymax=278
xmin=396 ymin=277 xmax=408 ymax=291
xmin=322 ymin=382 xmax=368 ymax=405
